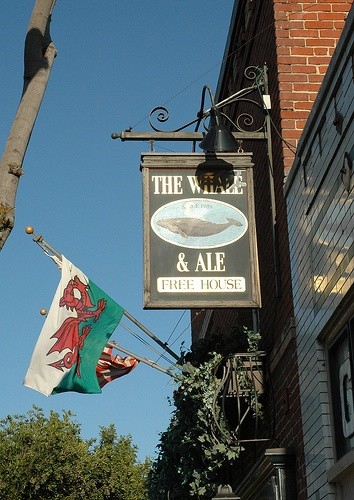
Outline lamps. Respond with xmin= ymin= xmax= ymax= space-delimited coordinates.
xmin=196 ymin=82 xmax=241 ymax=156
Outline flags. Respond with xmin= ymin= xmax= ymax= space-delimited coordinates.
xmin=22 ymin=254 xmax=124 ymax=397
xmin=97 ymin=341 xmax=140 ymax=390
